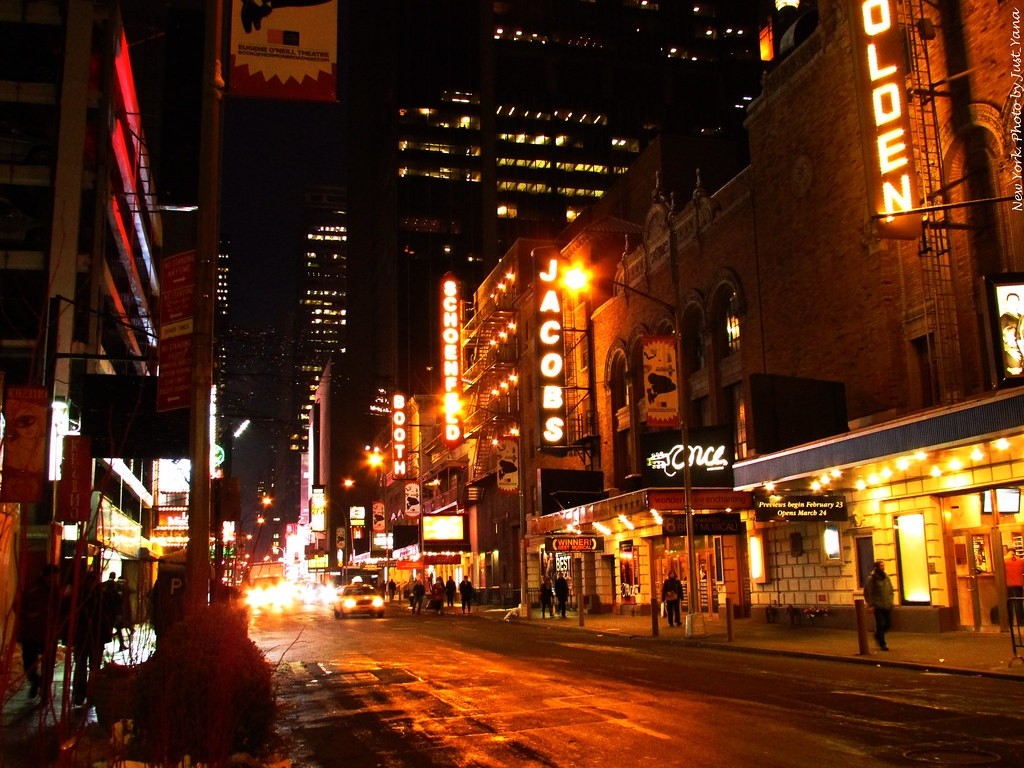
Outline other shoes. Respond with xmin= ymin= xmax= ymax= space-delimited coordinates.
xmin=28 ymin=678 xmax=39 ymax=698
xmin=438 ymin=610 xmax=441 ymax=614
xmin=417 ymin=609 xmax=420 ymax=614
xmin=119 ymin=644 xmax=129 ymax=650
xmin=542 ymin=613 xmax=545 ymax=618
xmin=467 ymin=611 xmax=471 ymax=615
xmin=874 ymin=633 xmax=877 ymax=641
xmin=463 ymin=610 xmax=465 ymax=615
xmin=74 ymin=682 xmax=87 ymax=705
xmin=881 ymin=647 xmax=888 ymax=650
xmin=562 ymin=615 xmax=566 ymax=618
xmin=670 ymin=624 xmax=674 ymax=628
xmin=550 ymin=613 xmax=555 ymax=618
xmin=412 ymin=609 xmax=415 ymax=614
xmin=677 ymin=622 xmax=682 ymax=626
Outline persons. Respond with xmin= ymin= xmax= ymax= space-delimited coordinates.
xmin=389 ymin=579 xmax=396 ymax=599
xmin=540 ymin=576 xmax=555 ymax=618
xmin=661 ymin=570 xmax=684 ymax=626
xmin=446 ymin=576 xmax=457 ymax=607
xmin=405 ymin=572 xmax=445 ymax=615
xmin=1003 ymin=545 xmax=1024 ymax=626
xmin=554 ymin=572 xmax=569 ymax=617
xmin=459 ymin=576 xmax=473 ymax=614
xmin=14 ymin=562 xmax=135 ymax=710
xmin=864 ymin=561 xmax=894 ymax=651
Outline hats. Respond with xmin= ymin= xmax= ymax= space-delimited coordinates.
xmin=40 ymin=564 xmax=60 ymax=577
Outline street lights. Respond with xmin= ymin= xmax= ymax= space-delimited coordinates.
xmin=313 ymin=496 xmax=349 ymax=584
xmin=563 ymin=266 xmax=697 ymax=639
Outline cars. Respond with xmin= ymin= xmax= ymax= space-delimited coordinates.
xmin=333 ymin=583 xmax=385 ymax=619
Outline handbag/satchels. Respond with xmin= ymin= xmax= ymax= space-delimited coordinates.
xmin=661 ymin=602 xmax=667 ymax=618
xmin=665 ymin=591 xmax=678 ymax=602
